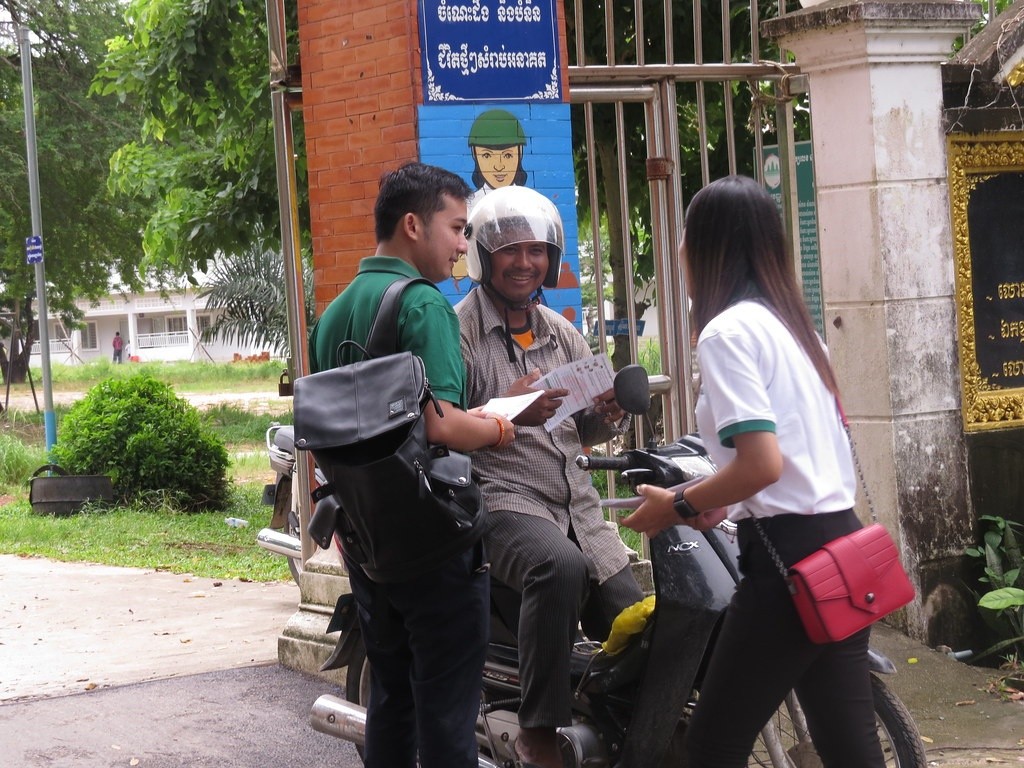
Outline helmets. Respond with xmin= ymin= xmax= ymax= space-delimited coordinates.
xmin=466 ymin=184 xmax=564 ymax=289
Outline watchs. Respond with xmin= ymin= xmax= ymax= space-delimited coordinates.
xmin=673 ymin=487 xmax=701 ymax=519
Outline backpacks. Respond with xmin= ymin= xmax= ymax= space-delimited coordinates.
xmin=291 ymin=275 xmax=492 ymax=582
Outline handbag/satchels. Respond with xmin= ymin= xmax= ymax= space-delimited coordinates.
xmin=792 ymin=527 xmax=916 ymax=646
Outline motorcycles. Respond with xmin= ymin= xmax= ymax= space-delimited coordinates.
xmin=297 ymin=359 xmax=930 ymax=768
xmin=259 ymin=356 xmax=301 ymax=576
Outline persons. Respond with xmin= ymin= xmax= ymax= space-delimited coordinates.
xmin=112 ymin=331 xmax=123 ymax=364
xmin=452 ymin=186 xmax=645 ymax=768
xmin=309 ymin=159 xmax=515 ymax=768
xmin=618 ymin=175 xmax=888 ymax=768
xmin=125 ymin=340 xmax=130 ymax=358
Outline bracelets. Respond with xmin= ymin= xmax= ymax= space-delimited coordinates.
xmin=487 ymin=416 xmax=505 ymax=449
